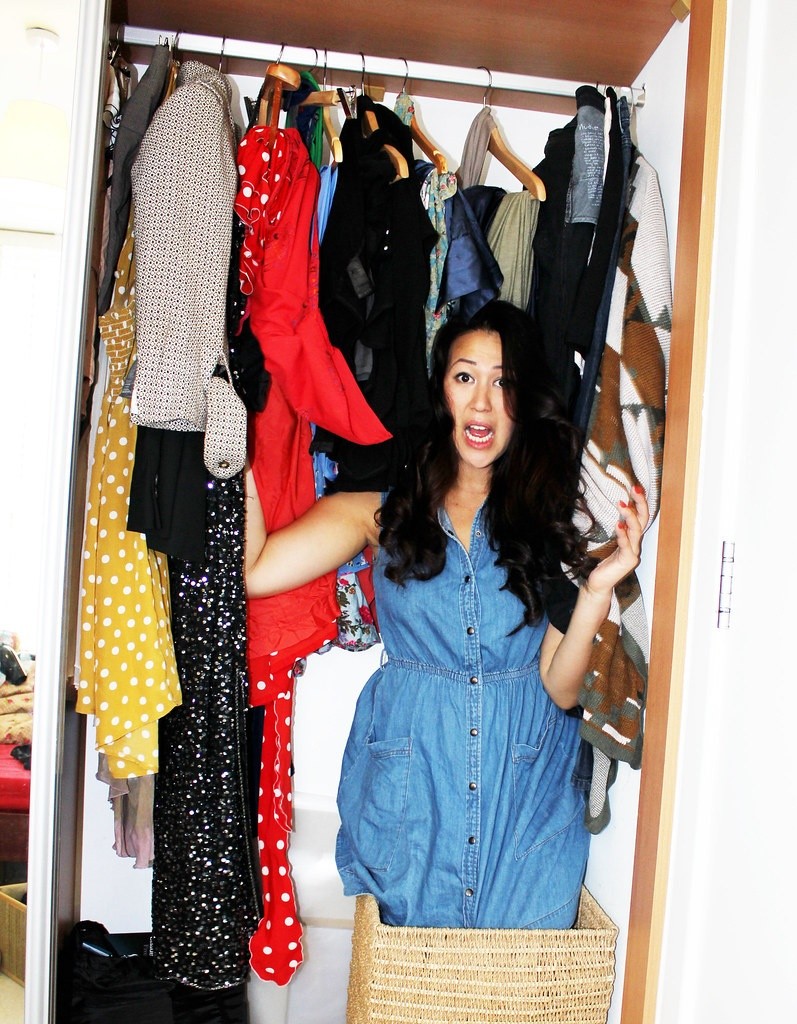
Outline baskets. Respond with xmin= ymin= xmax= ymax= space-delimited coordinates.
xmin=345 ymin=882 xmax=620 ymax=1024
xmin=0 ymin=882 xmax=27 ymax=990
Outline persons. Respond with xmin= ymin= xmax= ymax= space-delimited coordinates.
xmin=243 ymin=302 xmax=649 ymax=930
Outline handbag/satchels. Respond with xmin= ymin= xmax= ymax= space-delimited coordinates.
xmin=58 ymin=920 xmax=175 ymax=1024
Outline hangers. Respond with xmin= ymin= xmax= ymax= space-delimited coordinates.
xmin=102 ymin=29 xmax=635 ymax=203
